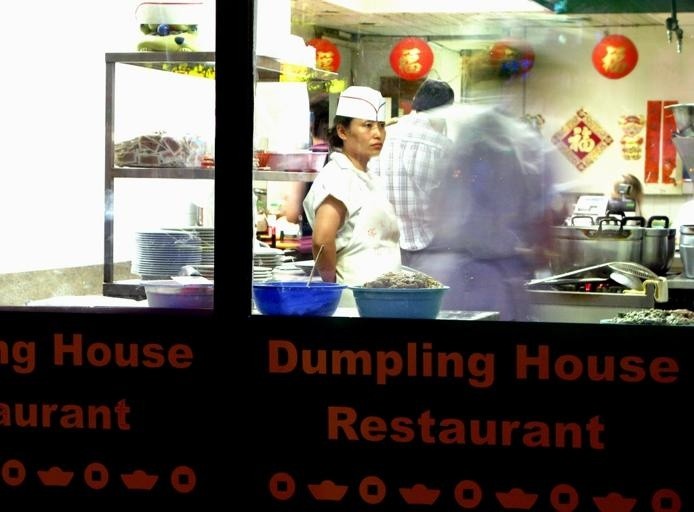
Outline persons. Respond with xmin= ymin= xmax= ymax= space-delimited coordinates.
xmin=365 ymin=78 xmax=453 ymax=264
xmin=428 ymin=41 xmax=577 ymax=323
xmin=286 ymin=96 xmax=330 ymax=252
xmin=304 ymin=115 xmax=402 ymax=287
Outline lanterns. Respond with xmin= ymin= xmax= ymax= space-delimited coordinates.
xmin=592 ymin=35 xmax=640 ymax=79
xmin=307 ymin=39 xmax=340 ymax=74
xmin=489 ymin=36 xmax=535 ymax=75
xmin=388 ymin=38 xmax=432 ymax=81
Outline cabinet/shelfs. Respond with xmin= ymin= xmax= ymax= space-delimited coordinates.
xmin=103 ymin=51 xmax=338 ymax=300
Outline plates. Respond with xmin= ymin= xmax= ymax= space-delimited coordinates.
xmin=294 ymin=259 xmax=321 ymax=277
xmin=131 ymin=227 xmax=285 ymax=279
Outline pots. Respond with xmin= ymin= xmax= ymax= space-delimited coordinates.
xmin=548 ymin=210 xmax=694 ymax=280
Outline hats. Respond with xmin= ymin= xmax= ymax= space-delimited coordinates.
xmin=335 ymin=85 xmax=385 ymax=122
xmin=412 ymin=80 xmax=454 ymax=111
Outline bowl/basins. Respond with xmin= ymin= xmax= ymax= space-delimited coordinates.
xmin=348 ymin=283 xmax=451 ymax=319
xmin=138 ymin=278 xmax=214 ymax=310
xmin=254 ymin=280 xmax=347 ymax=316
xmin=268 ymin=151 xmax=329 ymax=172
